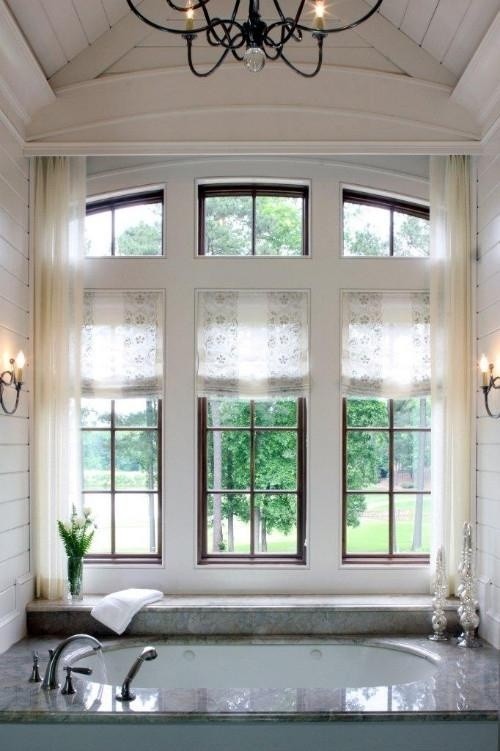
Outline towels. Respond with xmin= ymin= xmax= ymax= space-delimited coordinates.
xmin=91 ymin=587 xmax=163 ymax=638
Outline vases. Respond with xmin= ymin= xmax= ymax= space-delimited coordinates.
xmin=64 ymin=558 xmax=85 ymax=602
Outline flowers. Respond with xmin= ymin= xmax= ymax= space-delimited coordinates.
xmin=57 ymin=504 xmax=100 ymax=556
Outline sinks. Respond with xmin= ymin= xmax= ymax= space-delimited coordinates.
xmin=62 ymin=636 xmax=441 ymax=690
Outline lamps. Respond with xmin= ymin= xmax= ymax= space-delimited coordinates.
xmin=476 ymin=349 xmax=500 ymax=418
xmin=128 ymin=0 xmax=383 ymax=79
xmin=0 ymin=346 xmax=28 ymax=417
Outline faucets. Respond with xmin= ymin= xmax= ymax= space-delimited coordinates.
xmin=40 ymin=634 xmax=103 ymax=689
